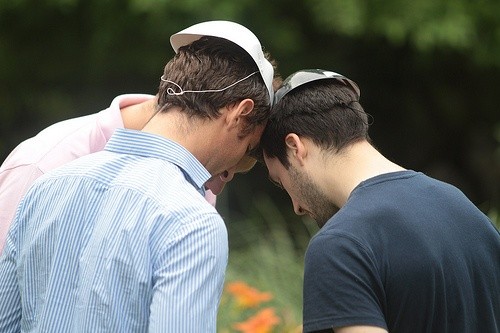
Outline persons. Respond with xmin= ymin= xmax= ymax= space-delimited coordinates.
xmin=0 ymin=21 xmax=274 ymax=332
xmin=260 ymin=69 xmax=499 ymax=333
xmin=0 ymin=46 xmax=287 ymax=255
